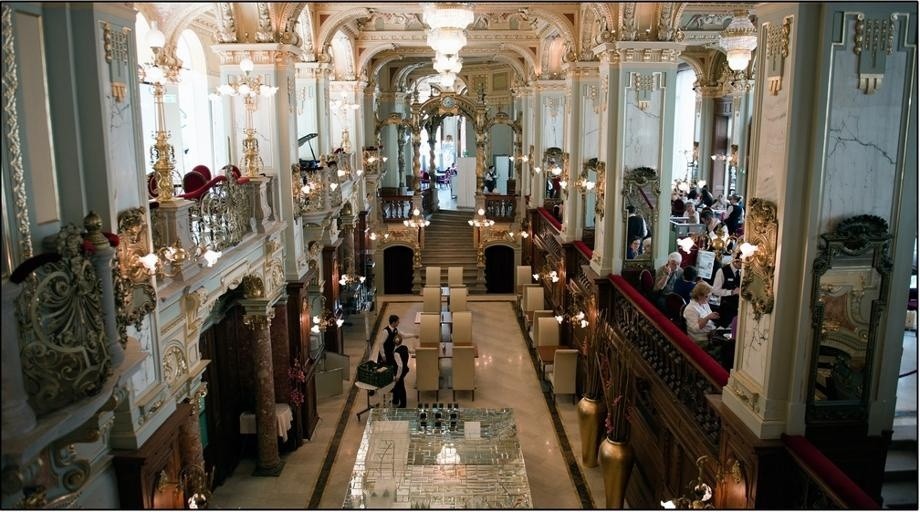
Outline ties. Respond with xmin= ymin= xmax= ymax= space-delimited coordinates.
xmin=735 ymin=269 xmax=740 ymax=288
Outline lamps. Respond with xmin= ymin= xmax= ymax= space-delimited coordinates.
xmin=140 ymin=28 xmax=279 ymax=201
xmin=157 ymin=463 xmax=219 ymax=509
xmin=116 ymin=207 xmax=225 ymax=331
xmin=676 ymin=196 xmax=780 ymax=321
xmin=301 ymin=294 xmax=345 ymax=335
xmin=418 ymin=2 xmax=476 ymax=90
xmin=660 ymin=454 xmax=744 ymax=509
xmin=719 ymin=10 xmax=759 ymax=72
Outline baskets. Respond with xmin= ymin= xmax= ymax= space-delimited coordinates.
xmin=357 ymin=360 xmax=394 ymax=388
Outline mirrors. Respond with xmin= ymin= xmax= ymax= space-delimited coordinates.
xmin=540 ymin=145 xmax=661 ymax=272
xmin=806 ymin=214 xmax=895 ymax=427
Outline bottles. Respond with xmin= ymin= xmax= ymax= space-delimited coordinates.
xmin=372 ymin=365 xmax=377 ymax=374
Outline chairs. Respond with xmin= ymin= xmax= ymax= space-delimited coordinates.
xmin=638 ymin=268 xmax=687 ymax=330
xmin=515 ymin=264 xmax=580 ymax=408
xmin=411 ymin=265 xmax=478 ymax=403
xmin=180 ymin=163 xmax=241 ymax=239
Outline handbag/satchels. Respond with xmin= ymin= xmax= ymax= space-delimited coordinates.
xmin=706 ymin=329 xmax=735 ymax=346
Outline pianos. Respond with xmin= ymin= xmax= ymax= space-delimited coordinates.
xmin=298 ymin=134 xmax=320 ymax=204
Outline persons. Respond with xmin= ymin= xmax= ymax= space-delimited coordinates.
xmin=377 ymin=315 xmax=419 ymax=364
xmin=625 ymin=181 xmax=745 ymax=370
xmin=546 ymin=159 xmax=564 ymax=221
xmin=485 ymin=166 xmax=497 ymax=192
xmin=392 ymin=334 xmax=409 ymax=409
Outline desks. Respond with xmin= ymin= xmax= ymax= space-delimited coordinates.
xmin=239 ymin=402 xmax=293 ymax=437
